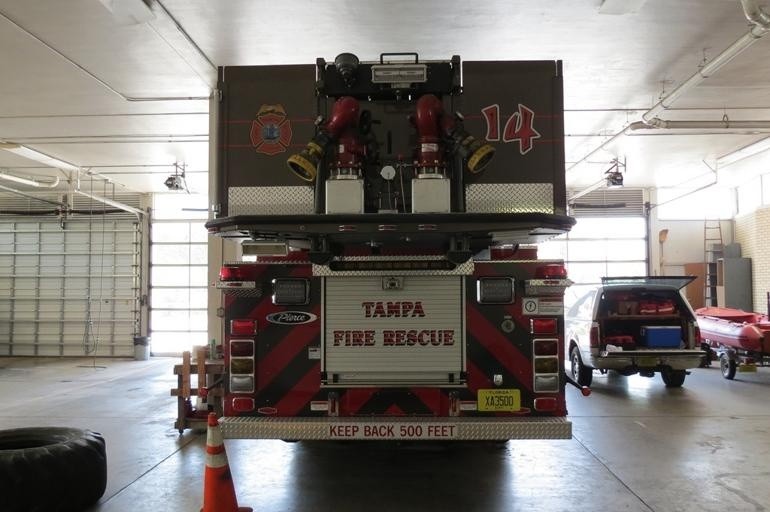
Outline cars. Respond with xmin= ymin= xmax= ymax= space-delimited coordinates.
xmin=564 ymin=276 xmax=706 ymax=387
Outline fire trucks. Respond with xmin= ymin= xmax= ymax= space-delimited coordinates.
xmin=198 ymin=54 xmax=592 ymax=446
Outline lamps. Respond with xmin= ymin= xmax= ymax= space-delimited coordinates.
xmin=605 ymin=158 xmax=624 ymax=187
xmin=164 ymin=162 xmax=185 ymax=190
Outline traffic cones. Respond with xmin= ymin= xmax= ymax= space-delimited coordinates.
xmin=198 ymin=414 xmax=254 ymax=512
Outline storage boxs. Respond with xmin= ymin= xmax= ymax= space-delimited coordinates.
xmin=614 ymin=300 xmax=639 ymax=315
xmin=641 ymin=325 xmax=681 ymax=348
